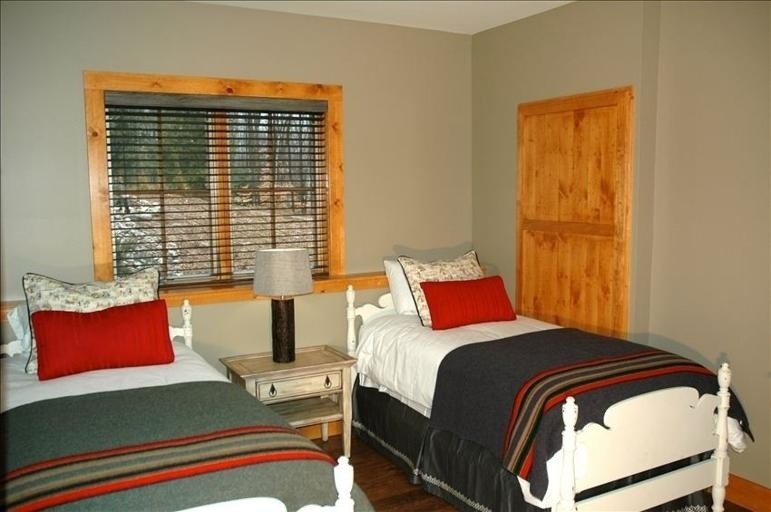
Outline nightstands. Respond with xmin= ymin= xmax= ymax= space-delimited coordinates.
xmin=219 ymin=346 xmax=356 ymax=460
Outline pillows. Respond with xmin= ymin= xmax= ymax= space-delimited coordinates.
xmin=383 ymin=260 xmax=415 ymax=317
xmin=31 ymin=300 xmax=174 ymax=380
xmin=7 ymin=305 xmax=30 ymax=354
xmin=420 ymin=276 xmax=518 ymax=329
xmin=399 ymin=251 xmax=483 ymax=326
xmin=21 ymin=268 xmax=160 ymax=374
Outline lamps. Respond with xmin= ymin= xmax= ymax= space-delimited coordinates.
xmin=254 ymin=248 xmax=314 ymax=363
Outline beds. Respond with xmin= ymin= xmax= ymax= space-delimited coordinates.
xmin=347 ymin=287 xmax=753 ymax=512
xmin=0 ymin=298 xmax=373 ymax=512
